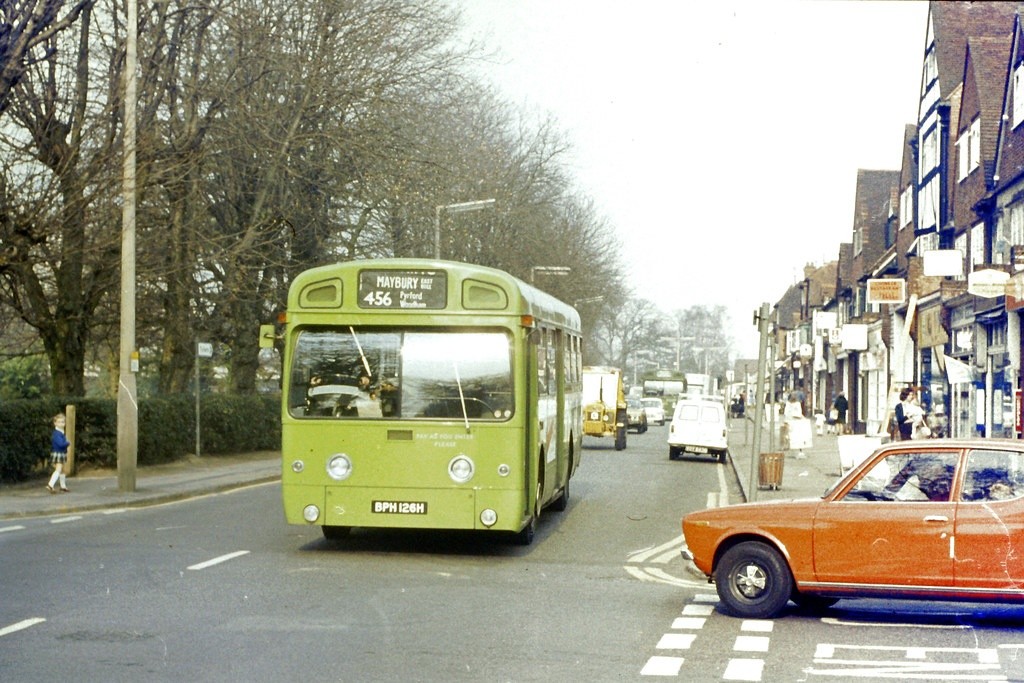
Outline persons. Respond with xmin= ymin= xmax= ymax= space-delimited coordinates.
xmin=895 ymin=388 xmax=923 ymax=440
xmin=833 ymin=392 xmax=848 ymax=435
xmin=46 ymin=413 xmax=70 ymax=494
xmin=815 ymin=409 xmax=827 ymax=436
xmin=731 ymin=389 xmax=754 ymax=418
xmin=826 ymin=405 xmax=837 ymax=434
xmin=764 ymin=387 xmax=807 ymax=418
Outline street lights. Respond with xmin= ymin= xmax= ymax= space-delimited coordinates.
xmin=529 ymin=267 xmax=572 ymax=289
xmin=433 ymin=198 xmax=496 ymax=259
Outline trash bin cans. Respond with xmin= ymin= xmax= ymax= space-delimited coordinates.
xmin=759 ymin=452 xmax=784 ymax=486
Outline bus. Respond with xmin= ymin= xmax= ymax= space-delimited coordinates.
xmin=259 ymin=256 xmax=584 ymax=545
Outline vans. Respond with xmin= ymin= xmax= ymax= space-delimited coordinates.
xmin=667 ymin=400 xmax=731 ymax=464
xmin=625 ymin=372 xmax=759 ymax=411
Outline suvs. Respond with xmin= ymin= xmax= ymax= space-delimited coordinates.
xmin=580 ymin=364 xmax=628 ymax=452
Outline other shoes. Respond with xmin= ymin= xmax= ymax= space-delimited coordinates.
xmin=59 ymin=488 xmax=70 ymax=492
xmin=45 ymin=485 xmax=56 ymax=494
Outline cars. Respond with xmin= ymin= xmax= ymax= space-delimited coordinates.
xmin=624 ymin=398 xmax=648 ymax=434
xmin=639 ymin=397 xmax=668 ymax=427
xmin=681 ymin=437 xmax=1024 ymax=620
